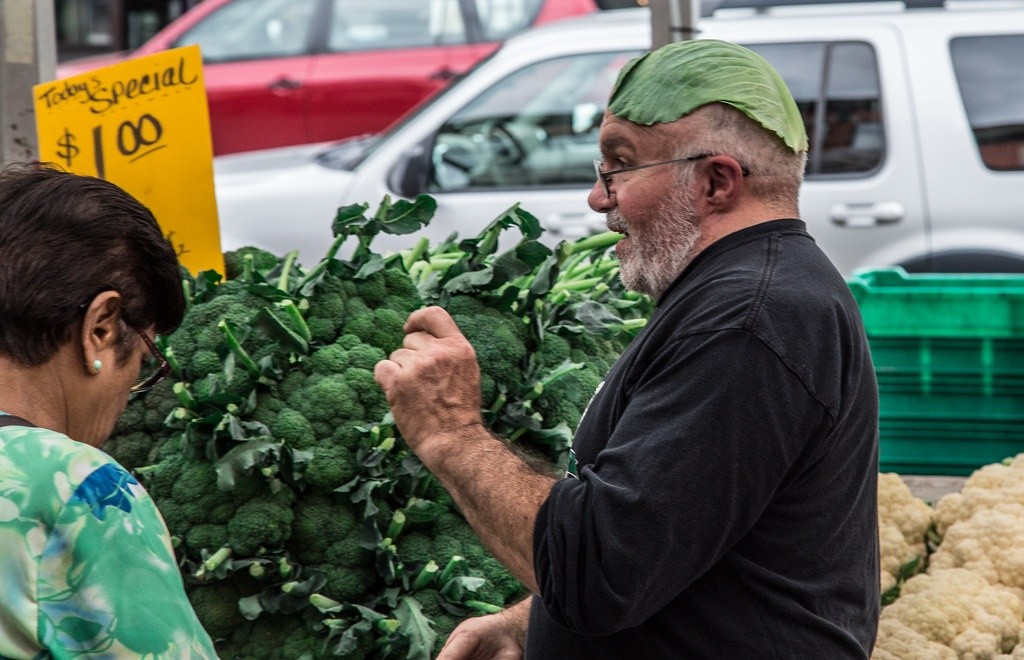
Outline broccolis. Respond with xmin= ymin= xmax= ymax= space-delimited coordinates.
xmin=869 ymin=449 xmax=1024 ymax=660
xmin=96 ymin=194 xmax=656 ymax=660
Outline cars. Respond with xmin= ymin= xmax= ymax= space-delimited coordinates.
xmin=55 ymin=0 xmax=629 ymax=159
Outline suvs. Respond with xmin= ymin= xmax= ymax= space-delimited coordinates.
xmin=212 ymin=0 xmax=1024 ymax=280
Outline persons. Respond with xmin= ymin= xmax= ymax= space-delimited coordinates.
xmin=0 ymin=161 xmax=222 ymax=660
xmin=373 ymin=38 xmax=882 ymax=660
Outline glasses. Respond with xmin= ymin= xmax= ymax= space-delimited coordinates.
xmin=81 ymin=297 xmax=171 ymax=393
xmin=595 ymin=150 xmax=750 ymax=200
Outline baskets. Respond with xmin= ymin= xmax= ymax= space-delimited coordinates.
xmin=849 ymin=263 xmax=1024 ymax=475
